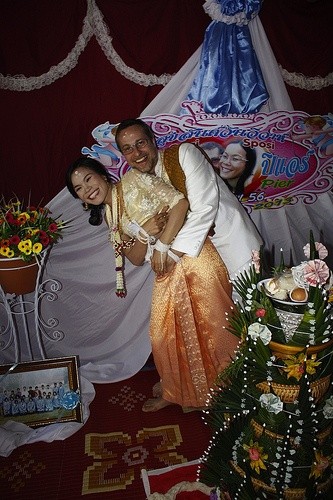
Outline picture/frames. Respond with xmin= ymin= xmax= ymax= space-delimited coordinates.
xmin=0 ymin=355 xmax=83 ymax=430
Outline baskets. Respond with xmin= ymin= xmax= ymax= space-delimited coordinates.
xmin=232 ymin=374 xmax=333 ymax=500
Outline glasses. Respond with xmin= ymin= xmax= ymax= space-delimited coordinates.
xmin=122 ymin=138 xmax=149 ymax=155
xmin=219 ymin=154 xmax=248 ymax=164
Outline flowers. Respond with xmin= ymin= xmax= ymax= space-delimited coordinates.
xmin=0 ymin=190 xmax=71 ymax=270
xmin=243 ymin=242 xmax=333 ymax=474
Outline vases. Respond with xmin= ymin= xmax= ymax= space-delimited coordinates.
xmin=0 ymin=254 xmax=43 ymax=296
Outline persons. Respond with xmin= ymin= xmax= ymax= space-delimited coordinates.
xmin=2 ymin=381 xmax=65 ymax=416
xmin=114 ymin=118 xmax=263 ymax=313
xmin=66 ymin=157 xmax=243 ymax=415
xmin=199 ymin=138 xmax=256 ymax=196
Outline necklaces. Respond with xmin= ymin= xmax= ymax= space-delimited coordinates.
xmin=103 ymin=184 xmax=157 ymax=299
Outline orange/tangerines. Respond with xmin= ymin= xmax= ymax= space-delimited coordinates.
xmin=291 ymin=288 xmax=305 ymax=301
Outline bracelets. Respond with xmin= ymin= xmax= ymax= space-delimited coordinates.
xmin=167 ymin=250 xmax=180 ymax=263
xmin=155 ymin=239 xmax=169 ymax=252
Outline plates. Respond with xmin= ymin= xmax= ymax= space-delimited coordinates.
xmin=256 ymin=278 xmax=306 ymax=305
